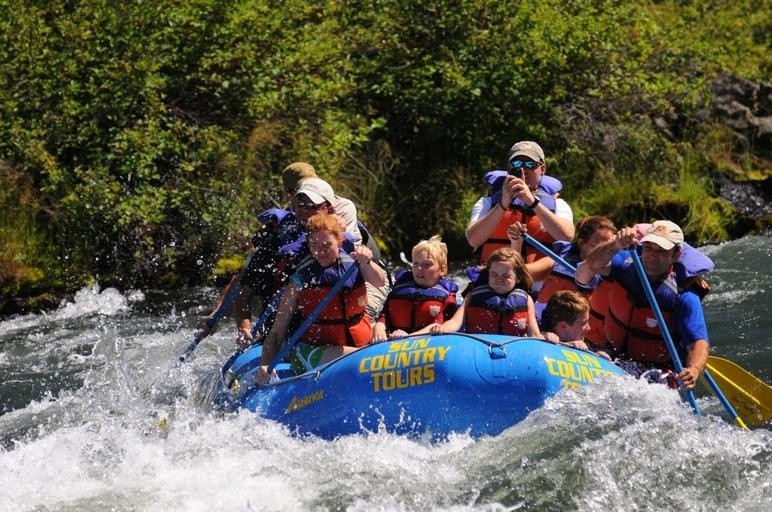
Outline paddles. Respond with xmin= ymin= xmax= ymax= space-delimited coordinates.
xmin=520 ymin=233 xmax=772 ymax=427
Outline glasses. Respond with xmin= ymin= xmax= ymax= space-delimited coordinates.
xmin=510 ymin=160 xmax=538 ymax=170
xmin=295 ymin=198 xmax=316 ymax=208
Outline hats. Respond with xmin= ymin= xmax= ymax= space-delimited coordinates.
xmin=638 ymin=220 xmax=684 ymax=251
xmin=293 ymin=177 xmax=336 ymax=208
xmin=282 ymin=161 xmax=320 ymax=188
xmin=507 ymin=141 xmax=545 ymax=164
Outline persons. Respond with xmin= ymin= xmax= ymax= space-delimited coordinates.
xmin=234 ymin=160 xmax=387 ymax=391
xmin=574 ymin=220 xmax=716 ymax=390
xmin=542 ymin=290 xmax=612 ymax=361
xmin=430 ymin=247 xmax=560 ymax=345
xmin=464 ymin=140 xmax=576 ymax=266
xmin=534 ymin=216 xmax=617 ymax=328
xmin=370 ymin=234 xmax=460 ymax=345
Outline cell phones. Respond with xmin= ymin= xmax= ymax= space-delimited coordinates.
xmin=510 ymin=168 xmax=521 ymax=178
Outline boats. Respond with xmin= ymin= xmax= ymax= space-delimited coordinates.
xmin=205 ymin=331 xmax=637 ymax=448
